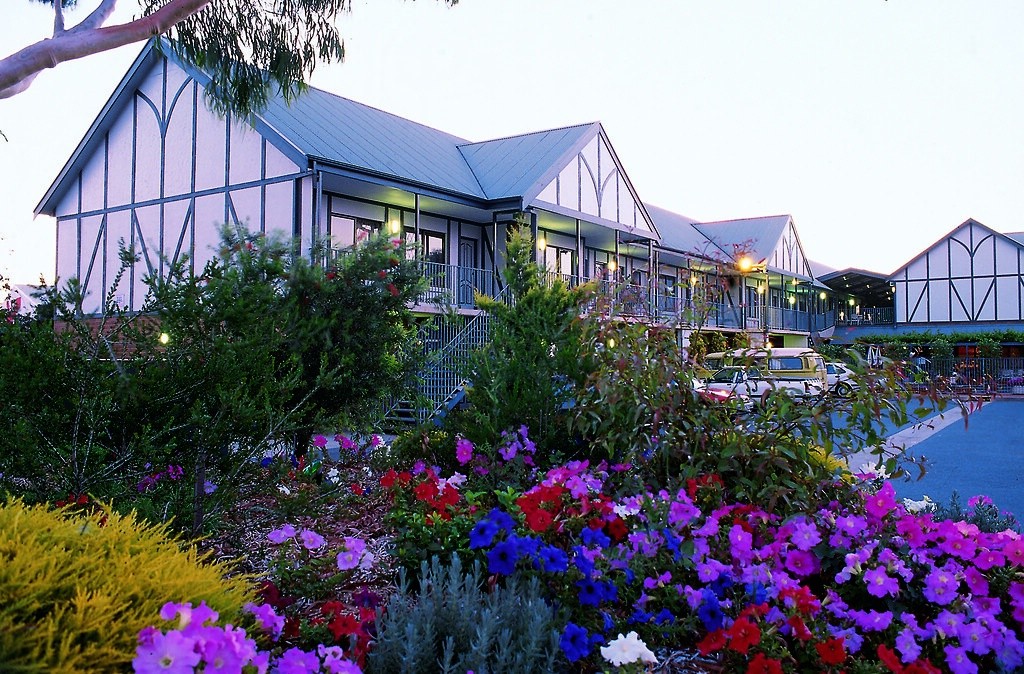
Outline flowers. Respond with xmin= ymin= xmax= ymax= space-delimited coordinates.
xmin=1007 ymin=377 xmax=1024 ymax=386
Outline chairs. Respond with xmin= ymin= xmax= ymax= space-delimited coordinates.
xmin=851 ymin=314 xmax=860 ymax=326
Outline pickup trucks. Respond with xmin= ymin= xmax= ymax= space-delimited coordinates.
xmin=703 ymin=365 xmax=823 ymax=403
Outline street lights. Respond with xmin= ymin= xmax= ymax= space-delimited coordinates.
xmin=741 ymin=260 xmax=769 ymax=349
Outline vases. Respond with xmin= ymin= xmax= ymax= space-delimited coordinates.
xmin=1012 ymin=386 xmax=1024 ymax=394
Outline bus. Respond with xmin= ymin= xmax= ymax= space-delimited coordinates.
xmin=694 ymin=348 xmax=828 ymax=405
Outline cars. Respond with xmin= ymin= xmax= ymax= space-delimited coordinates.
xmin=683 ymin=372 xmax=738 ymax=423
xmin=825 ymin=361 xmax=861 ymax=398
